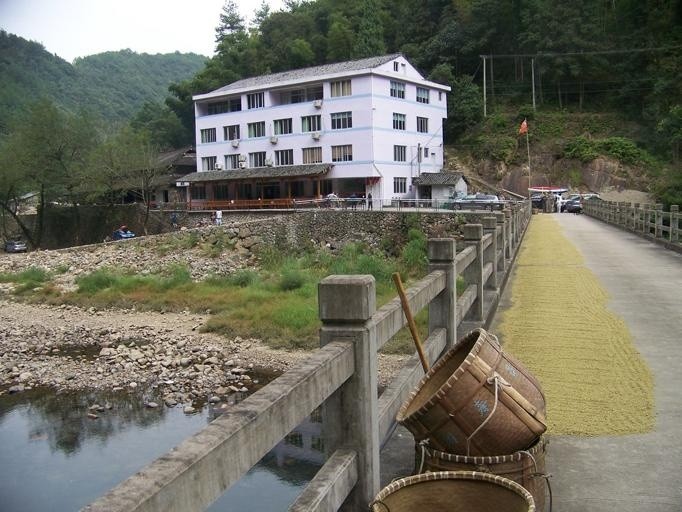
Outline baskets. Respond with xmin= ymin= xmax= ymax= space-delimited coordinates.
xmin=371 ymin=327 xmax=555 ymax=512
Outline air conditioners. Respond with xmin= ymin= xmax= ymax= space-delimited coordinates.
xmin=270 ymin=137 xmax=278 ymax=143
xmin=312 ymin=132 xmax=320 ymax=140
xmin=239 ymin=162 xmax=246 ymax=168
xmin=214 ymin=163 xmax=222 ymax=170
xmin=232 ymin=140 xmax=239 ymax=147
xmin=238 ymin=155 xmax=246 ymax=162
xmin=264 ymin=159 xmax=272 ymax=166
xmin=314 ymin=100 xmax=322 ymax=107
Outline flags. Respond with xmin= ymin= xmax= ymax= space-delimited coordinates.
xmin=519 ymin=120 xmax=528 ymax=134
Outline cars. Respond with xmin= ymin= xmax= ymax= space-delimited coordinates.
xmin=450 ymin=188 xmax=600 ymax=213
xmin=6 ymin=240 xmax=28 ymax=253
xmin=113 ymin=226 xmax=136 ymax=240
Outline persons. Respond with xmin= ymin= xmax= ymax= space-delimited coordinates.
xmin=320 ymin=192 xmax=372 ymax=211
xmin=215 ymin=207 xmax=223 ymax=227
